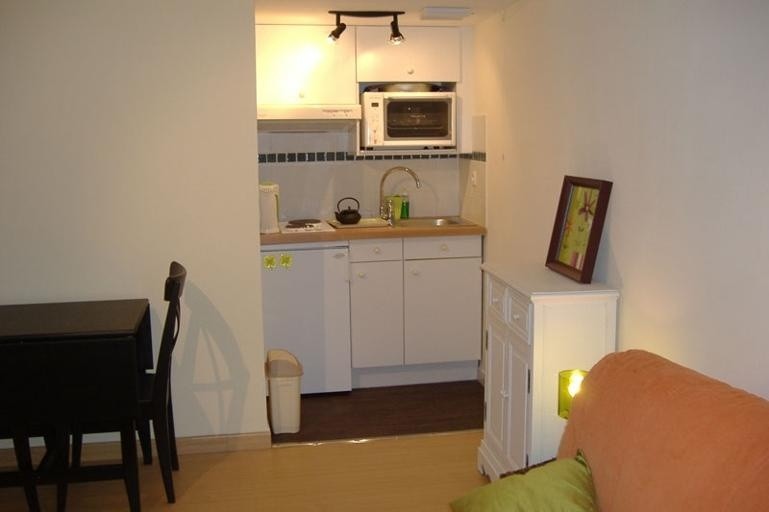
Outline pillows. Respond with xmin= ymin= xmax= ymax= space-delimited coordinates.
xmin=448 ymin=447 xmax=599 ymax=512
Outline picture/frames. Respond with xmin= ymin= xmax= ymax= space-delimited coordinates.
xmin=545 ymin=175 xmax=613 ymax=284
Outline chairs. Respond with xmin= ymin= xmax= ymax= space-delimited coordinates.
xmin=54 ymin=260 xmax=181 ymax=512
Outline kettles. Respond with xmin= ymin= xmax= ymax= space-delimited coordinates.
xmin=259 ymin=182 xmax=280 ymax=234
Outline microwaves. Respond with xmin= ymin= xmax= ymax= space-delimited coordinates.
xmin=360 ymin=91 xmax=458 ymax=151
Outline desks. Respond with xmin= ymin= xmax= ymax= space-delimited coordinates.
xmin=0 ymin=301 xmax=153 ymax=512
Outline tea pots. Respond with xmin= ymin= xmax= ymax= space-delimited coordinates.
xmin=335 ymin=197 xmax=361 ymax=225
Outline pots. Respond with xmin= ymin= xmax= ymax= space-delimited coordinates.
xmin=364 ymin=82 xmax=447 ymax=92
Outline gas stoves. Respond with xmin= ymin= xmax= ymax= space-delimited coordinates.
xmin=281 ymin=217 xmax=337 ymax=234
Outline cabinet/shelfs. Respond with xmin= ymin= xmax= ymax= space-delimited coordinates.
xmin=477 ymin=263 xmax=619 ymax=484
xmin=357 ymin=25 xmax=461 ymax=84
xmin=253 ymin=24 xmax=356 ymax=107
xmin=259 ymin=228 xmax=483 ymax=395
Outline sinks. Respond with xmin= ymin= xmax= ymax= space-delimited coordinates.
xmin=384 ymin=216 xmax=470 ymax=226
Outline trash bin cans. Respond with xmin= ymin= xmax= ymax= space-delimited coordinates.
xmin=265 ymin=350 xmax=304 ymax=435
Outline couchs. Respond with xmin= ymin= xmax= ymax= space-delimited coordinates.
xmin=554 ymin=347 xmax=768 ymax=512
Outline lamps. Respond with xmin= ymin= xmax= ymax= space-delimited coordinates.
xmin=558 ymin=369 xmax=589 ymax=419
xmin=328 ymin=11 xmax=405 ymax=46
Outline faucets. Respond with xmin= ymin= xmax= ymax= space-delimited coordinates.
xmin=379 ymin=166 xmax=421 ymax=218
